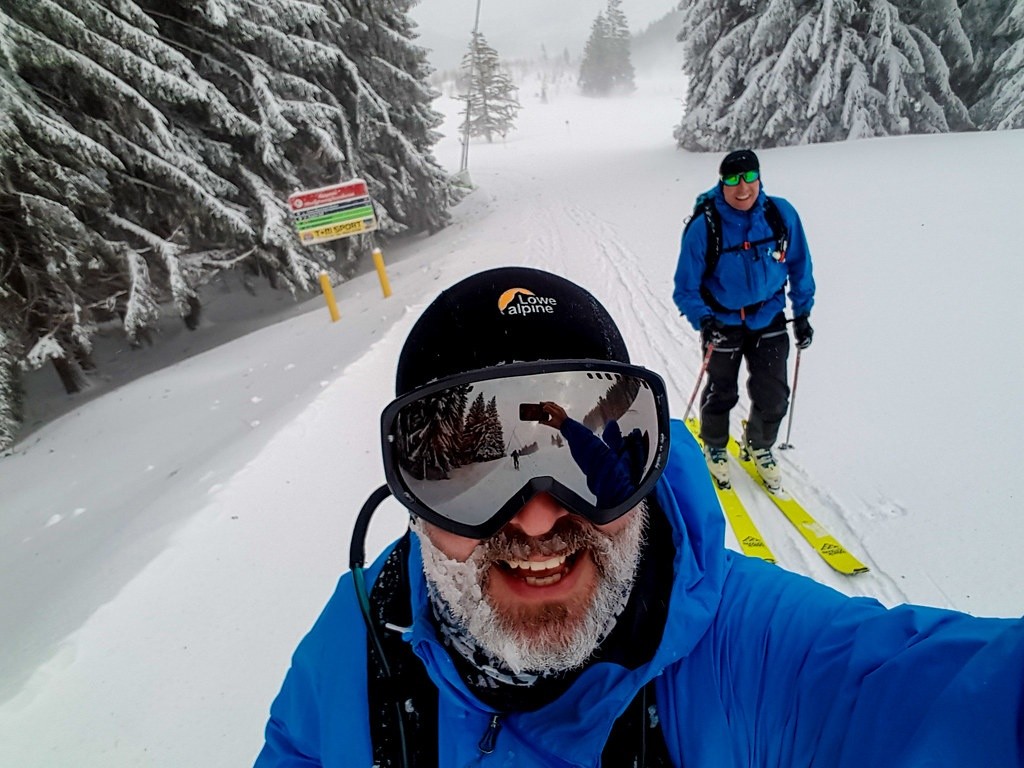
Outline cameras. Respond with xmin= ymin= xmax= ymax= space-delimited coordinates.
xmin=519 ymin=403 xmax=550 ymax=421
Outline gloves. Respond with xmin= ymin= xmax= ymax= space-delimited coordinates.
xmin=699 ymin=317 xmax=729 ymax=352
xmin=793 ymin=315 xmax=813 ymax=350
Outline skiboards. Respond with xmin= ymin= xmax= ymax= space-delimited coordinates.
xmin=684 ymin=416 xmax=871 ymax=578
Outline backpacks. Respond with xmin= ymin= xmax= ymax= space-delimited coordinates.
xmin=616 ymin=428 xmax=649 ymax=487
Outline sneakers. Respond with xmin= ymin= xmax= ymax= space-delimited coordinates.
xmin=742 ymin=432 xmax=783 ymax=492
xmin=704 ymin=442 xmax=729 ymax=484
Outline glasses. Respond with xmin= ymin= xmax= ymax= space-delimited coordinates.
xmin=720 ymin=170 xmax=759 ymax=186
xmin=380 ymin=359 xmax=671 ymax=539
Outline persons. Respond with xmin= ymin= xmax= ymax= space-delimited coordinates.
xmin=255 ymin=267 xmax=1024 ymax=768
xmin=511 ymin=449 xmax=519 ymax=468
xmin=674 ymin=150 xmax=816 ymax=492
xmin=539 ymin=402 xmax=649 ymax=509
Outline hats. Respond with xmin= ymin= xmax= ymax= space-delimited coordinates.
xmin=395 ymin=267 xmax=631 ymax=425
xmin=719 ymin=149 xmax=759 ymax=176
